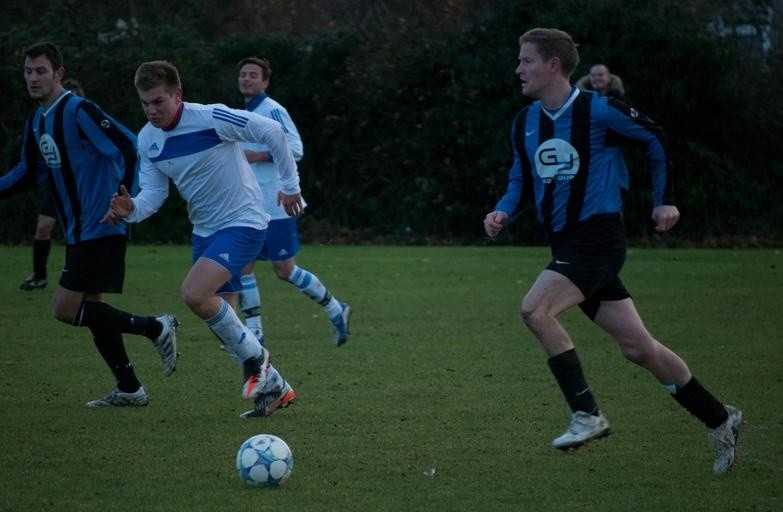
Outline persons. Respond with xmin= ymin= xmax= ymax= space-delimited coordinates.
xmin=109 ymin=60 xmax=307 ymax=419
xmin=0 ymin=42 xmax=179 ymax=408
xmin=574 ymin=64 xmax=636 ymax=110
xmin=19 ymin=78 xmax=86 ymax=292
xmin=225 ymin=58 xmax=352 ymax=348
xmin=483 ymin=29 xmax=743 ymax=475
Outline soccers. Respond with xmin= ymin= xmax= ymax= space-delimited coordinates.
xmin=237 ymin=434 xmax=293 ymax=487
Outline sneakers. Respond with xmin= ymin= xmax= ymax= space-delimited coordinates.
xmin=239 ymin=345 xmax=270 ymax=401
xmin=19 ymin=274 xmax=48 ymax=289
xmin=329 ymin=300 xmax=353 ymax=348
xmin=237 ymin=377 xmax=298 ymax=418
xmin=708 ymin=401 xmax=742 ymax=477
xmin=83 ymin=383 xmax=149 ymax=409
xmin=550 ymin=410 xmax=611 ymax=449
xmin=153 ymin=311 xmax=182 ymax=377
